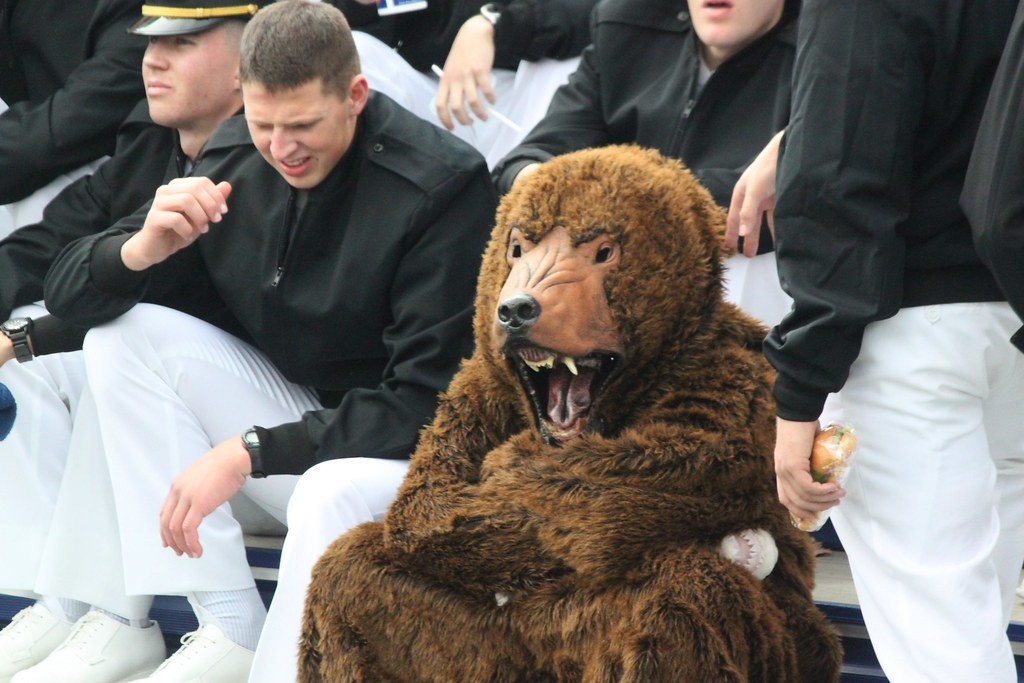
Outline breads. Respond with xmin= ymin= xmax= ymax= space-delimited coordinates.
xmin=791 ymin=425 xmax=857 ymax=531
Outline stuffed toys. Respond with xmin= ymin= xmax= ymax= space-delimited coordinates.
xmin=298 ymin=143 xmax=846 ymax=683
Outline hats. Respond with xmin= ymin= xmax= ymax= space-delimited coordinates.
xmin=125 ymin=0 xmax=276 ymax=36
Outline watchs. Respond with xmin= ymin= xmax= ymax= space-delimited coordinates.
xmin=242 ymin=428 xmax=264 ymax=478
xmin=0 ymin=317 xmax=32 ymax=363
xmin=480 ymin=2 xmax=501 ymax=25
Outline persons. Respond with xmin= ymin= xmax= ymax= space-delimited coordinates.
xmin=0 ymin=0 xmax=1024 ymax=683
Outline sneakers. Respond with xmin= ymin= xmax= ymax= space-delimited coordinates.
xmin=10 ymin=611 xmax=166 ymax=683
xmin=128 ymin=624 xmax=257 ymax=683
xmin=0 ymin=603 xmax=77 ymax=683
